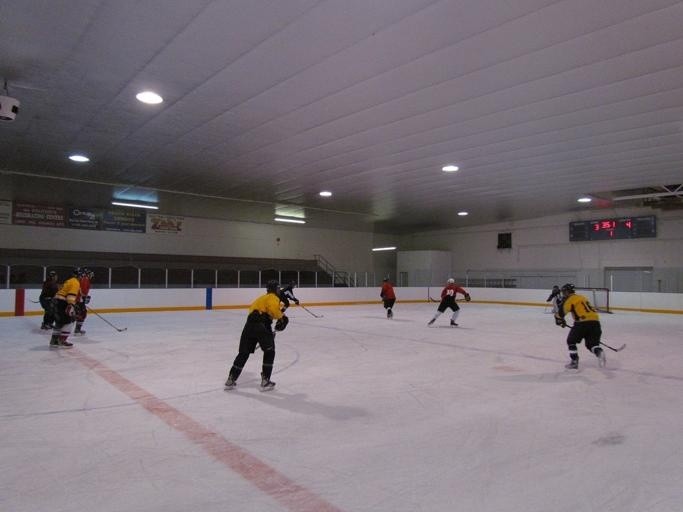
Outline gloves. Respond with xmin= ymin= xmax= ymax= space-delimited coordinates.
xmin=275 ymin=315 xmax=288 ymax=331
xmin=64 ymin=305 xmax=76 ymax=317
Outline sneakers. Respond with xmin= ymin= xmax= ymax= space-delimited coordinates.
xmin=224 ymin=378 xmax=276 ymax=389
xmin=563 ymin=348 xmax=605 ymax=371
xmin=41 ymin=324 xmax=86 ymax=348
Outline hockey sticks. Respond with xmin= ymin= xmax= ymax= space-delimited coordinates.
xmin=86 ymin=304 xmax=128 ymax=331
xmin=566 ymin=323 xmax=626 ymax=352
xmin=298 ymin=303 xmax=323 ymax=319
xmin=429 ymin=296 xmax=467 ymax=303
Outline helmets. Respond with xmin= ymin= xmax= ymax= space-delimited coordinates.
xmin=48 ymin=267 xmax=95 ymax=281
xmin=447 ymin=278 xmax=455 ymax=284
xmin=266 ymin=279 xmax=297 ymax=296
xmin=562 ymin=283 xmax=575 ymax=296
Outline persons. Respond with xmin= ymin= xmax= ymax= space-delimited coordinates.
xmin=548 ymin=283 xmax=616 ymax=372
xmin=225 ymin=279 xmax=286 ymax=391
xmin=428 ymin=278 xmax=471 ymax=327
xmin=380 ymin=278 xmax=396 ymax=319
xmin=273 ymin=280 xmax=298 ymax=316
xmin=38 ymin=266 xmax=94 ymax=347
